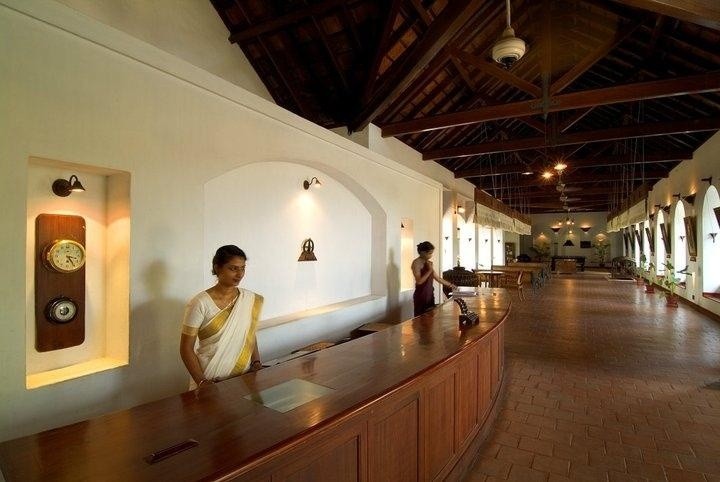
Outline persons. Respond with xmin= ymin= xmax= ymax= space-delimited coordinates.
xmin=180 ymin=245 xmax=264 ymax=391
xmin=412 ymin=241 xmax=457 ymax=317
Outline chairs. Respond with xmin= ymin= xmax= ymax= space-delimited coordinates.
xmin=500 ymin=269 xmax=525 ymax=302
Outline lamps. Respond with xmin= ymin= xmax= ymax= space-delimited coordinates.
xmin=52 ymin=175 xmax=86 ymax=197
xmin=490 ymin=0 xmax=528 ymax=63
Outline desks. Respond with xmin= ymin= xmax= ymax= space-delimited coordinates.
xmin=552 ymin=255 xmax=585 ymax=272
xmin=477 ymin=271 xmax=506 ymax=288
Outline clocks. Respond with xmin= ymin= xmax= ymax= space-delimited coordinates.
xmin=49 ymin=239 xmax=87 ymax=273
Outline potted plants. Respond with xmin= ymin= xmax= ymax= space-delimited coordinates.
xmin=637 ymin=253 xmax=692 ymax=308
xmin=592 ymin=241 xmax=611 ymax=267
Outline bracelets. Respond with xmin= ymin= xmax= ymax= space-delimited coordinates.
xmin=448 ymin=283 xmax=452 ymax=288
xmin=253 ymin=359 xmax=260 ymax=366
xmin=198 ymin=378 xmax=207 ymax=386
